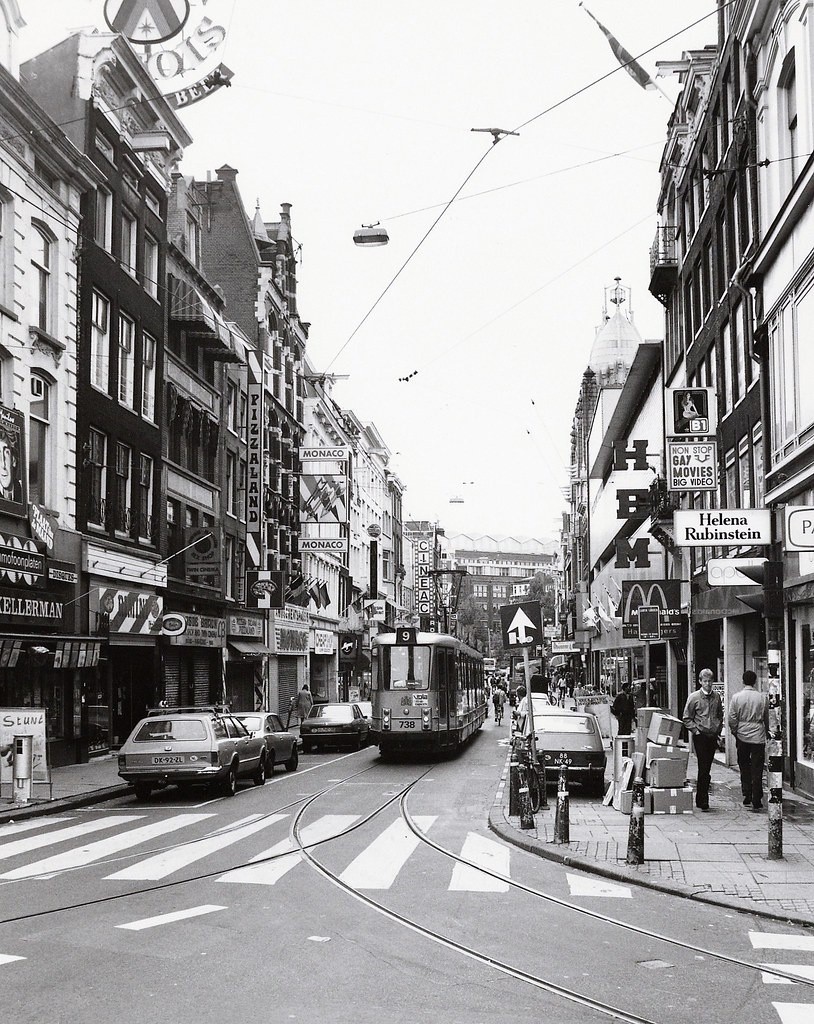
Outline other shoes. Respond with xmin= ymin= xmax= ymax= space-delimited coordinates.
xmin=753 ymin=801 xmax=763 ymax=809
xmin=743 ymin=794 xmax=753 ymax=806
xmin=698 ymin=801 xmax=710 ymax=812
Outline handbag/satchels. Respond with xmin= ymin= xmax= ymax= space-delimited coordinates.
xmin=493 ymin=694 xmax=499 ymax=704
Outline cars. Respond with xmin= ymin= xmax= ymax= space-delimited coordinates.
xmin=519 ymin=711 xmax=608 ymax=795
xmin=300 ymin=704 xmax=372 ymax=752
xmin=224 ymin=713 xmax=299 ymax=778
xmin=510 ymin=694 xmax=568 ymax=743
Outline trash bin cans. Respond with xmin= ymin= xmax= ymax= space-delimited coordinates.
xmin=12 ymin=734 xmax=34 ymax=804
xmin=614 ymin=736 xmax=636 ymax=811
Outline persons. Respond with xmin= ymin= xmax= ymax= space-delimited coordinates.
xmin=490 ymin=675 xmax=507 ymax=722
xmin=683 ymin=668 xmax=724 ymax=812
xmin=613 ymin=682 xmax=658 ymax=735
xmin=547 ymin=667 xmax=602 ymax=705
xmin=728 ymin=668 xmax=770 ymax=811
xmin=296 ymin=684 xmax=313 ymax=724
xmin=601 ymin=672 xmax=613 ymax=695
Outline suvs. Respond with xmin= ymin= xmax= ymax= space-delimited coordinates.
xmin=118 ymin=709 xmax=268 ymax=803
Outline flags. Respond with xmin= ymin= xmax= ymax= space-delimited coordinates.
xmin=352 ymin=598 xmax=362 ymax=614
xmin=583 ymin=581 xmax=622 ymax=627
xmin=290 ymin=576 xmax=311 ymax=607
xmin=365 ymin=604 xmax=377 ymax=620
xmin=309 ymin=581 xmax=321 ymax=609
xmin=320 ymin=583 xmax=331 ymax=610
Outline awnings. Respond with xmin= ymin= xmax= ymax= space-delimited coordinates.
xmin=228 ymin=641 xmax=278 ymax=657
xmin=205 ymin=333 xmax=246 ymax=363
xmin=385 ymin=600 xmax=409 ymax=622
xmin=356 ymin=650 xmax=371 ymax=671
xmin=170 ymin=278 xmax=215 ymax=332
xmin=188 ymin=305 xmax=230 ymax=349
xmin=549 ymin=656 xmax=562 ymax=667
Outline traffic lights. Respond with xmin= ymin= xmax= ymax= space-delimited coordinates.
xmin=734 ymin=560 xmax=771 ymax=617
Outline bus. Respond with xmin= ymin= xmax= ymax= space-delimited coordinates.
xmin=483 ymin=658 xmax=496 ymax=672
xmin=371 ymin=631 xmax=488 ymax=757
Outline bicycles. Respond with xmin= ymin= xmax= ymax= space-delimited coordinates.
xmin=495 ymin=706 xmax=502 ymax=726
xmin=511 ymin=733 xmax=546 ymax=813
xmin=545 ymin=691 xmax=557 ymax=706
